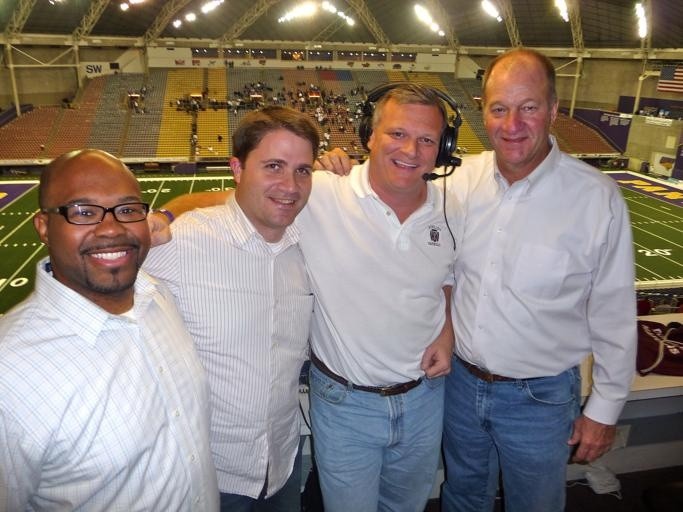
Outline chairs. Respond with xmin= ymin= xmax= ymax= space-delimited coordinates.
xmin=0 ymin=64 xmax=623 ymax=163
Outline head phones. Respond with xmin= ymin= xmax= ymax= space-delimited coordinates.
xmin=358 ymin=83 xmax=462 ymax=168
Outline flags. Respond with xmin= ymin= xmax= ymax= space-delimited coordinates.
xmin=656 ymin=67 xmax=682 ymax=93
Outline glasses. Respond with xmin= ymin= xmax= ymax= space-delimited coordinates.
xmin=42 ymin=203 xmax=149 ymax=226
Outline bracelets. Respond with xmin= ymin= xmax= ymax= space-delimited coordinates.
xmin=153 ymin=209 xmax=175 ymax=224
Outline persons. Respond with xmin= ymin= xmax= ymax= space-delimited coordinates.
xmin=143 ymin=79 xmax=459 ymax=511
xmin=126 ymin=66 xmax=375 ymax=163
xmin=310 ymin=46 xmax=639 ymax=511
xmin=0 ymin=146 xmax=225 ymax=511
xmin=132 ymin=104 xmax=318 ymax=512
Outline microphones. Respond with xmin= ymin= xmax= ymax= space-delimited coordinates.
xmin=422 ymin=157 xmax=461 ymax=181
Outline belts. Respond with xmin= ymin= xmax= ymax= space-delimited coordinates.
xmin=454 ymin=358 xmax=532 ymax=383
xmin=309 ymin=356 xmax=422 ymax=396
